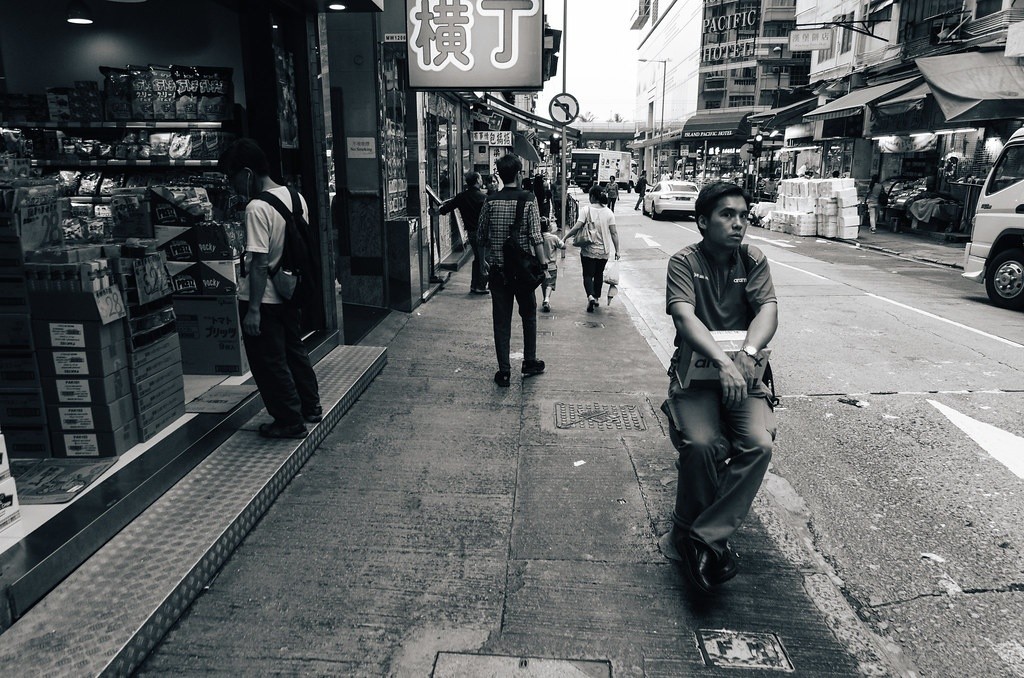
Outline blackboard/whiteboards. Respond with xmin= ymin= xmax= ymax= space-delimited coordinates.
xmin=454 ymin=208 xmax=469 ymax=252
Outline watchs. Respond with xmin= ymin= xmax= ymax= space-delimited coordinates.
xmin=740 ymin=346 xmax=758 ymax=358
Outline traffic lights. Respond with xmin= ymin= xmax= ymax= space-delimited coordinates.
xmin=753 ymin=133 xmax=764 ymax=158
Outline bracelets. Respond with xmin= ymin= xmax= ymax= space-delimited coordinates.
xmin=540 ymin=263 xmax=548 ymax=270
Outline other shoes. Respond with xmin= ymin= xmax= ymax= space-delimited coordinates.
xmin=468 ymin=289 xmax=489 ymax=295
xmin=870 ymin=228 xmax=876 ymax=233
xmin=542 ymin=300 xmax=551 ymax=312
xmin=587 ymin=297 xmax=595 ymax=312
xmin=595 ymin=302 xmax=600 ymax=307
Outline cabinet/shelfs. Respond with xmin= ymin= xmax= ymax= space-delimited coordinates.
xmin=0 ymin=121 xmax=223 ymax=202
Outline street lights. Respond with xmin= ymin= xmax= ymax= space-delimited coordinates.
xmin=638 ymin=58 xmax=667 ymax=183
xmin=769 ymin=42 xmax=785 ymax=190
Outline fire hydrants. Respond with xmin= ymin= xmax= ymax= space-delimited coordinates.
xmin=657 ymin=406 xmax=733 ymax=563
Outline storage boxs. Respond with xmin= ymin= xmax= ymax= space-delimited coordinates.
xmin=0 ymin=186 xmax=250 ymax=533
xmin=770 ymin=177 xmax=860 ymax=239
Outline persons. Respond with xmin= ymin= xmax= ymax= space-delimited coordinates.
xmin=478 ymin=154 xmax=552 ymax=388
xmin=665 ymin=180 xmax=780 ymax=591
xmin=634 ymin=170 xmax=652 ymax=209
xmin=605 ymin=175 xmax=620 ymax=213
xmin=521 ymin=169 xmax=562 ymax=234
xmin=539 ymin=216 xmax=567 ymax=312
xmin=589 ymin=180 xmax=607 ymax=204
xmin=485 ymin=173 xmax=500 ymax=195
xmin=215 ymin=136 xmax=324 ymax=439
xmin=561 ymin=185 xmax=621 ymax=313
xmin=866 ymin=174 xmax=884 ymax=234
xmin=750 ymin=161 xmax=840 ymax=191
xmin=440 ymin=171 xmax=489 ymax=296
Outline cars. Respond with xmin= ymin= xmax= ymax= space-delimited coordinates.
xmin=642 ymin=180 xmax=701 ymax=221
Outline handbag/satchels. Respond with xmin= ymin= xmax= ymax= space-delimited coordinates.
xmin=879 ymin=185 xmax=889 ymax=206
xmin=504 ymin=190 xmax=546 ymax=300
xmin=572 ymin=205 xmax=598 ymax=247
xmin=603 ymin=256 xmax=617 ymax=305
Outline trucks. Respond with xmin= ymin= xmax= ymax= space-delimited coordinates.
xmin=962 ymin=125 xmax=1024 ymax=311
xmin=569 ymin=148 xmax=640 ymax=194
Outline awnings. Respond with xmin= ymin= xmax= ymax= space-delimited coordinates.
xmin=682 ymin=111 xmax=753 ymax=139
xmin=801 ymin=74 xmax=921 ymax=122
xmin=921 ymin=7 xmax=969 ymax=41
xmin=486 ymin=92 xmax=581 ymax=137
xmin=625 ymin=127 xmax=683 ymax=149
xmin=747 ymin=96 xmax=818 ymax=123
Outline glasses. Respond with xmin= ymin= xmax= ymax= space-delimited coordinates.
xmin=520 ymin=170 xmax=525 ymax=175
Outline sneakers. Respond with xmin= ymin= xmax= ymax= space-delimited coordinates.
xmin=259 ymin=422 xmax=307 ymax=439
xmin=494 ymin=371 xmax=510 ymax=387
xmin=303 ymin=406 xmax=322 ymax=419
xmin=522 ymin=359 xmax=545 ymax=373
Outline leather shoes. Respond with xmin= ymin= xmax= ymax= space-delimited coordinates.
xmin=714 ymin=557 xmax=738 ymax=582
xmin=678 ymin=537 xmax=715 ymax=595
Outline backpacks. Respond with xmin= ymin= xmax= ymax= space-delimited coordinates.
xmin=635 ymin=177 xmax=643 ymax=193
xmin=252 ymin=185 xmax=322 ymax=303
